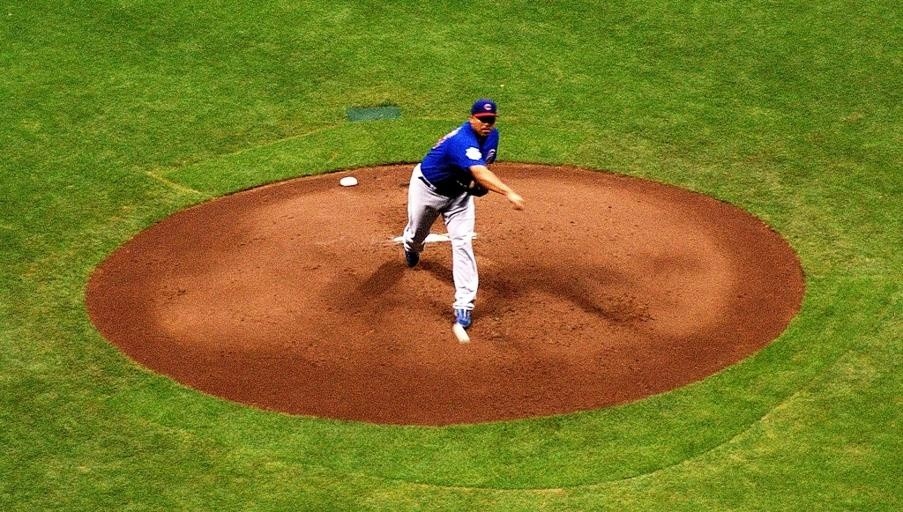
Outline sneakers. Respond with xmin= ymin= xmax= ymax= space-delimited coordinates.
xmin=404 ymin=250 xmax=420 ymax=268
xmin=454 ymin=308 xmax=472 ymax=329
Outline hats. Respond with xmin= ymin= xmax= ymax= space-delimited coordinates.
xmin=471 ymin=98 xmax=499 ymax=118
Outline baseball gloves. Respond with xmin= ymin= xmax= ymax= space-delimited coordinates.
xmin=454 ymin=174 xmax=488 ymax=197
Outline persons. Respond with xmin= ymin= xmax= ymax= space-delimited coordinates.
xmin=401 ymin=99 xmax=525 ymax=329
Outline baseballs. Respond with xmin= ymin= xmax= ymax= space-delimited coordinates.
xmin=340 ymin=177 xmax=357 ymax=186
